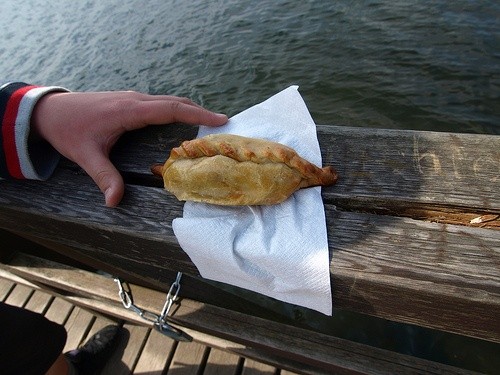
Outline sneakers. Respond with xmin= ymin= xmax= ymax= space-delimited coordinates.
xmin=68 ymin=325 xmax=124 ymax=373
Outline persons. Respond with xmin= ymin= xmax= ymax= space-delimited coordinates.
xmin=0 ymin=78 xmax=227 ymax=375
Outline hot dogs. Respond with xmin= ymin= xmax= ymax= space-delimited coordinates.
xmin=150 ymin=132 xmax=338 ymax=207
